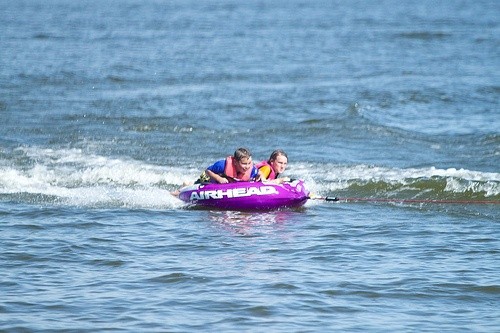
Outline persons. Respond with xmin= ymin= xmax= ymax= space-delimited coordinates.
xmin=171 ymin=147 xmax=290 ymax=196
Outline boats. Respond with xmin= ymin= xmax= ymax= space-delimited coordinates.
xmin=178 ymin=178 xmax=309 ymax=212
